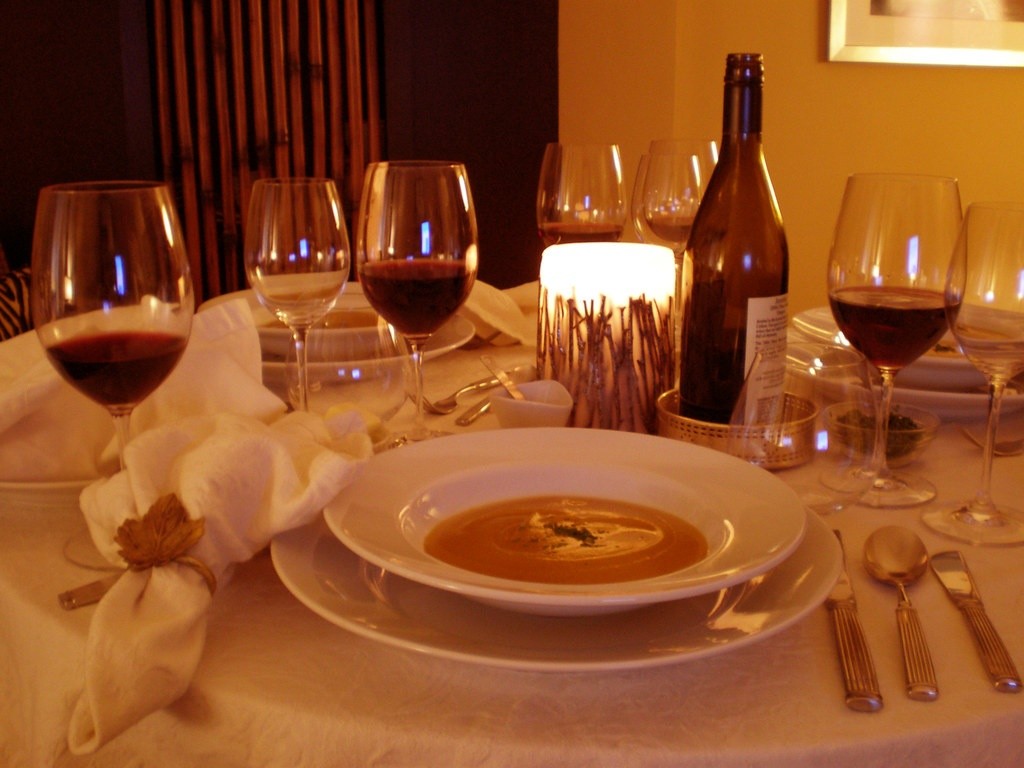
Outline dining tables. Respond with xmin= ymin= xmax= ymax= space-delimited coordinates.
xmin=1 ymin=291 xmax=1024 ymax=766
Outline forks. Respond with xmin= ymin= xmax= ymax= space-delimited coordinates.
xmin=406 ymin=364 xmax=533 ymax=414
xmin=956 ymin=426 xmax=1024 ymax=458
xmin=489 ymin=378 xmax=574 ymax=432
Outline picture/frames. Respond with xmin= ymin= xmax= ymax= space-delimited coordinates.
xmin=826 ymin=2 xmax=1024 ymax=64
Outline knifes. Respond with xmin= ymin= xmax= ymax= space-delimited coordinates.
xmin=813 ymin=529 xmax=883 ymax=715
xmin=926 ymin=549 xmax=1024 ymax=697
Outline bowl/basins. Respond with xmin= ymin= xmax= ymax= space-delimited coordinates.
xmin=819 ymin=400 xmax=942 ymax=468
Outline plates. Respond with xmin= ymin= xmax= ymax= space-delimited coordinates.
xmin=270 ymin=497 xmax=847 ymax=671
xmin=774 ymin=298 xmax=1024 ymax=426
xmin=316 ymin=427 xmax=810 ymax=616
xmin=198 ymin=281 xmax=475 ymax=389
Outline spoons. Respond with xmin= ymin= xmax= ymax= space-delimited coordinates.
xmin=864 ymin=526 xmax=940 ymax=703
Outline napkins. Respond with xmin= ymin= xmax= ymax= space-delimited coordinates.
xmin=468 ymin=279 xmax=534 ymax=347
xmin=66 ymin=296 xmax=372 ymax=756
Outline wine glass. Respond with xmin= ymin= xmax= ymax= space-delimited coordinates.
xmin=355 ymin=158 xmax=480 ymax=447
xmin=242 ymin=176 xmax=351 ymax=418
xmin=642 ymin=136 xmax=723 ymax=328
xmin=825 ymin=171 xmax=971 ymax=507
xmin=921 ymin=199 xmax=1024 ymax=544
xmin=30 ymin=182 xmax=197 ymax=573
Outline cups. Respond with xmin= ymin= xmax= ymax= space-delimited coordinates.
xmin=284 ymin=309 xmax=415 ymax=432
xmin=535 ymin=140 xmax=628 ymax=256
xmin=727 ymin=344 xmax=876 ymax=504
xmin=629 ymin=153 xmax=705 ymax=259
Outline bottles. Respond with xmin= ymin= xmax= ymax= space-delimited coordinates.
xmin=679 ymin=51 xmax=789 ymax=426
